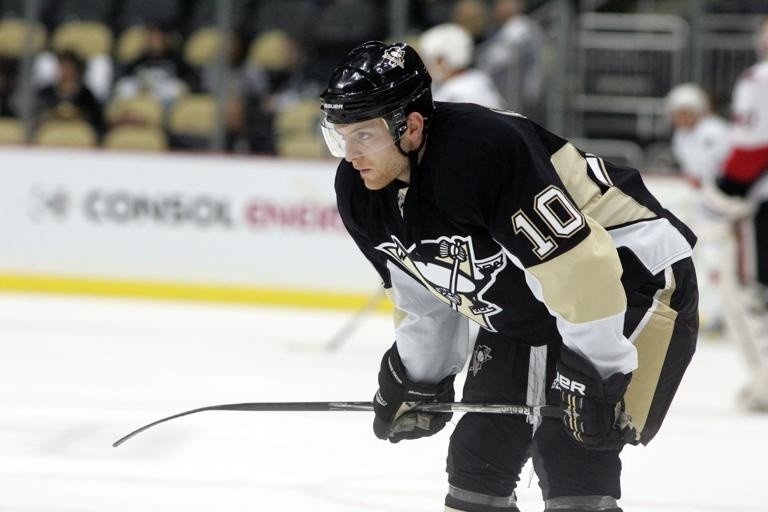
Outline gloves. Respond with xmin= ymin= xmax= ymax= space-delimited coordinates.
xmin=556 ymin=341 xmax=638 ymax=453
xmin=373 ymin=340 xmax=456 ymax=444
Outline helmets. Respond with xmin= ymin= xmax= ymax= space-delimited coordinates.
xmin=319 ymin=39 xmax=435 ymax=160
xmin=420 ymin=23 xmax=475 ymax=70
xmin=663 ymin=81 xmax=712 ymax=116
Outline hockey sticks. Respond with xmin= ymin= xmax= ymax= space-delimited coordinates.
xmin=112 ymin=402 xmax=563 ymax=448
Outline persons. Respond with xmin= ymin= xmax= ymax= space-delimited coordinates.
xmin=665 ymin=17 xmax=768 ymax=414
xmin=1 ymin=1 xmax=578 ymax=181
xmin=320 ymin=42 xmax=700 ymax=512
